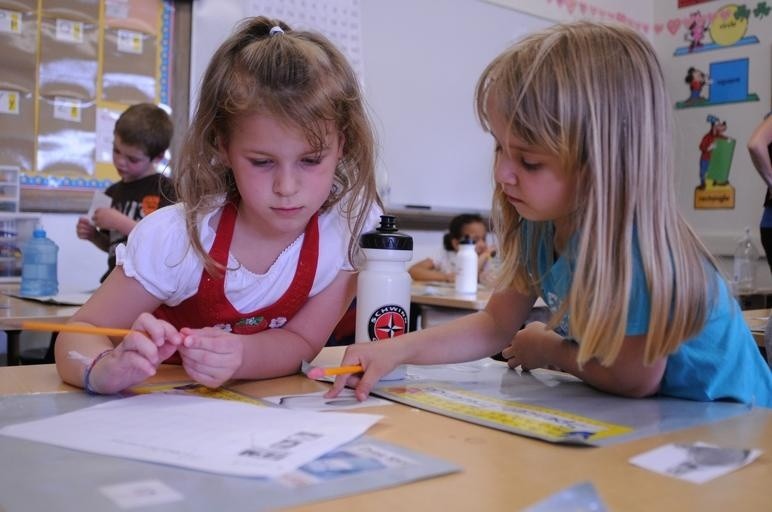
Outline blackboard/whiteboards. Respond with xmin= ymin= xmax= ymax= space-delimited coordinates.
xmin=189 ymin=0 xmax=571 ymax=219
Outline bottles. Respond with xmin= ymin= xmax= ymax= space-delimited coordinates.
xmin=453 ymin=233 xmax=480 ymax=295
xmin=733 ymin=226 xmax=759 ymax=291
xmin=354 ymin=213 xmax=411 ymax=382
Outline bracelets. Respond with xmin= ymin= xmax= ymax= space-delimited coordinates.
xmin=83 ymin=346 xmax=115 ymax=395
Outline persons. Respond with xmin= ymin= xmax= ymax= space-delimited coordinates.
xmin=746 ymin=110 xmax=772 ymax=269
xmin=76 ymin=102 xmax=177 ymax=283
xmin=53 ymin=14 xmax=389 ymax=398
xmin=322 ymin=17 xmax=772 ymax=409
xmin=408 ymin=212 xmax=498 ymax=330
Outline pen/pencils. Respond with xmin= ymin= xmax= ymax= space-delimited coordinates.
xmin=308 ymin=365 xmax=363 ymax=379
xmin=21 ymin=321 xmax=186 ymax=340
xmin=404 ymin=204 xmax=433 ymax=209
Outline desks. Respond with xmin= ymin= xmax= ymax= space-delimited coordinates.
xmin=1 ymin=344 xmax=771 ymax=511
xmin=412 ymin=289 xmax=545 ymax=329
xmin=1 ymin=283 xmax=81 ymax=365
